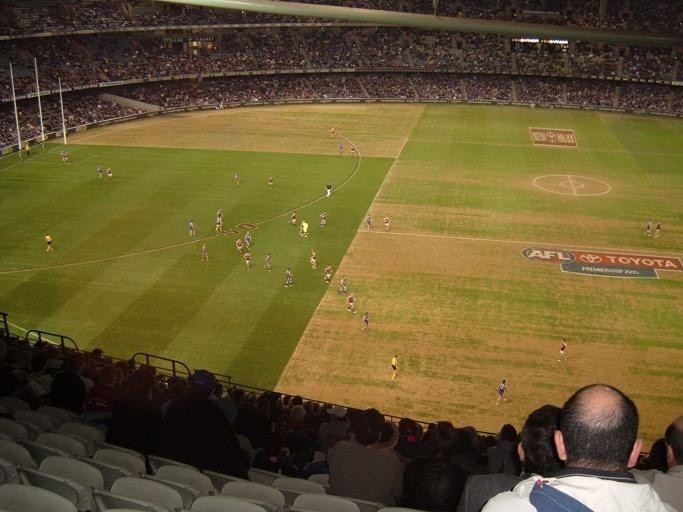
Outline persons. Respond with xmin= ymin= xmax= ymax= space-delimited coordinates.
xmin=233 ymin=170 xmax=239 ymax=186
xmin=267 ymin=176 xmax=273 ymax=190
xmin=324 ymin=183 xmax=332 ymax=198
xmin=187 ymin=208 xmax=373 ymax=334
xmin=96 ymin=165 xmax=103 ymax=178
xmin=3 ymin=326 xmax=683 ymax=512
xmin=644 ymin=220 xmax=654 ymax=236
xmin=655 ymin=223 xmax=662 ymax=238
xmin=106 ymin=168 xmax=112 ymax=178
xmin=44 ymin=233 xmax=54 ymax=253
xmin=384 ymin=216 xmax=390 ymax=232
xmin=0 ymin=2 xmax=683 ymax=156
